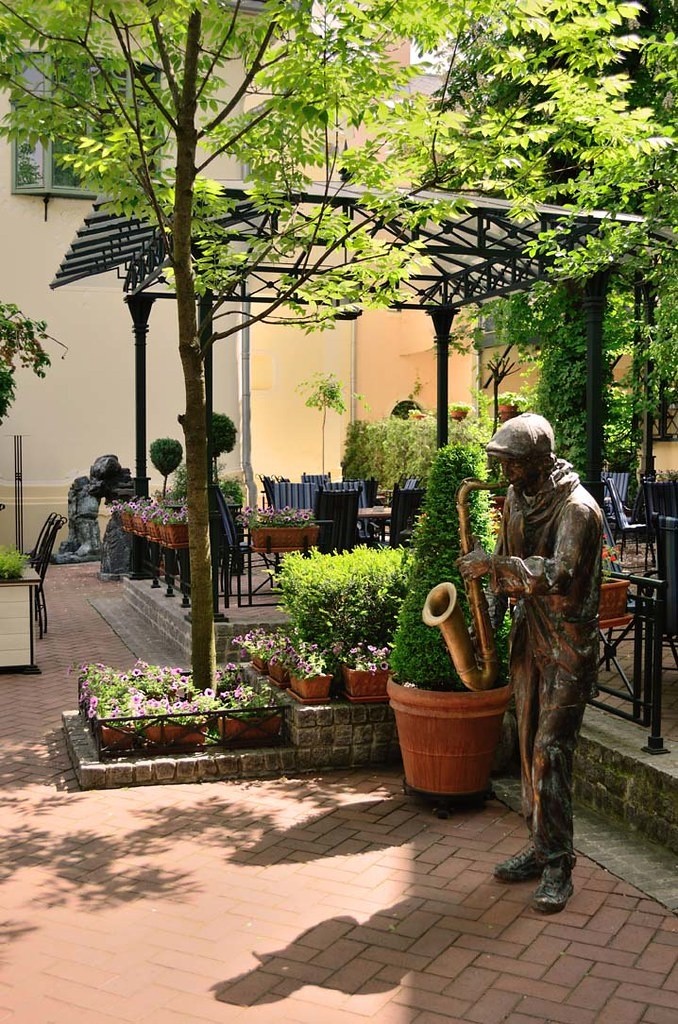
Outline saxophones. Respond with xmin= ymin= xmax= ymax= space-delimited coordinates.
xmin=421 ymin=475 xmax=512 ymax=691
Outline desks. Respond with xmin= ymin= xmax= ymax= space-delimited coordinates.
xmin=360 ymin=507 xmax=390 ymax=518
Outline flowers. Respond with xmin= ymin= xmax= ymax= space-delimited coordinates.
xmin=109 ymin=498 xmax=188 ymax=526
xmin=74 ymin=628 xmax=387 ymax=730
xmin=602 ymin=534 xmax=620 ymax=582
xmin=235 ymin=506 xmax=316 ymax=527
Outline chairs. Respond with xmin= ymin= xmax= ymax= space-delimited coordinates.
xmin=22 ymin=512 xmax=68 ymax=639
xmin=212 ymin=472 xmax=429 ymax=608
xmin=599 ymin=471 xmax=678 ymax=696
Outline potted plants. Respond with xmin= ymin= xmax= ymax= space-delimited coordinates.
xmin=388 ymin=440 xmax=511 ymax=810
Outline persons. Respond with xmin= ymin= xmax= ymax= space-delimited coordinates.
xmin=456 ymin=414 xmax=603 ymax=914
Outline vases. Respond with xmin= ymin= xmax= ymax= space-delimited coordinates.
xmin=143 ymin=725 xmax=207 ymax=746
xmin=252 ymin=527 xmax=319 ymax=547
xmin=287 ymin=671 xmax=332 ymax=700
xmin=120 ymin=513 xmax=188 ymax=545
xmin=268 ymin=660 xmax=287 ymax=683
xmin=599 ymin=578 xmax=630 ymax=620
xmin=100 ymin=725 xmax=133 ymax=752
xmin=216 ymin=716 xmax=281 ymax=744
xmin=341 ymin=664 xmax=388 ymax=697
